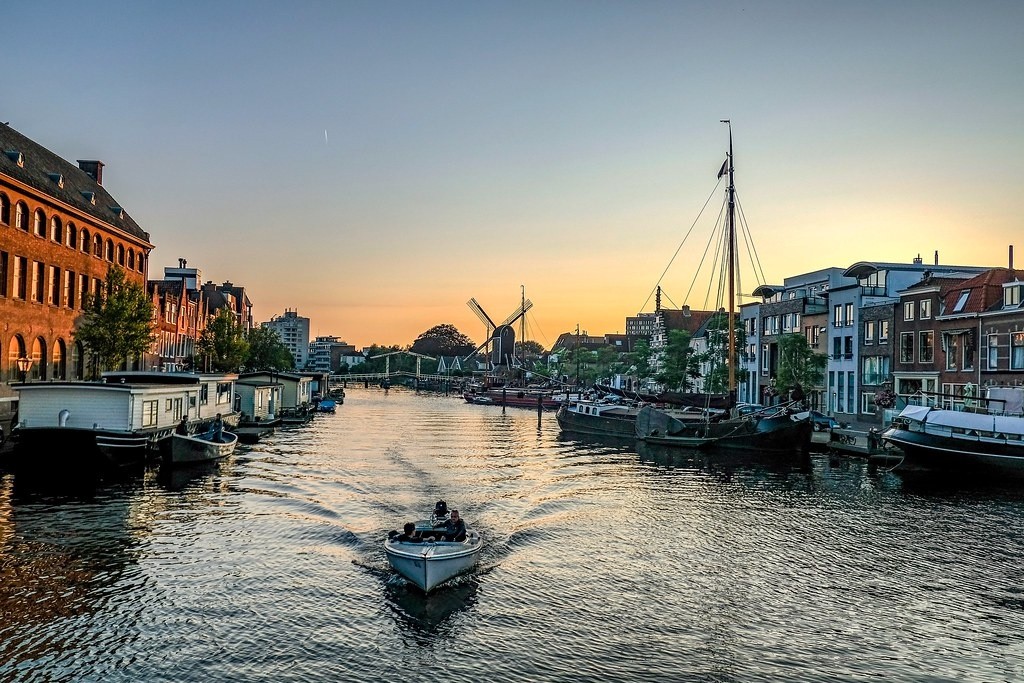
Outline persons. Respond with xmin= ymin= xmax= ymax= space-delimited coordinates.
xmin=394 ymin=522 xmax=435 ymax=543
xmin=433 ymin=509 xmax=467 ymax=541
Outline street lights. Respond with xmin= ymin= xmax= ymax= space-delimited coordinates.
xmin=17 ymin=355 xmax=33 ymax=388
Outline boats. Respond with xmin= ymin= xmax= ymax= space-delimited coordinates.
xmin=554 ymin=406 xmax=814 ymax=456
xmin=158 ymin=428 xmax=239 ymax=465
xmin=383 ymin=517 xmax=483 ymax=595
xmin=464 ymin=118 xmax=737 ymax=410
xmin=882 ymin=404 xmax=1024 ymax=474
xmin=321 ymin=401 xmax=337 ymax=414
xmin=327 ymin=387 xmax=344 ymax=403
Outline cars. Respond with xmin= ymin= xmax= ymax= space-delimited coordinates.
xmin=736 ymin=402 xmax=838 ymax=433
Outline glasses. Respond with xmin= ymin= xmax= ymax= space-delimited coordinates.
xmin=451 ymin=515 xmax=458 ymax=518
